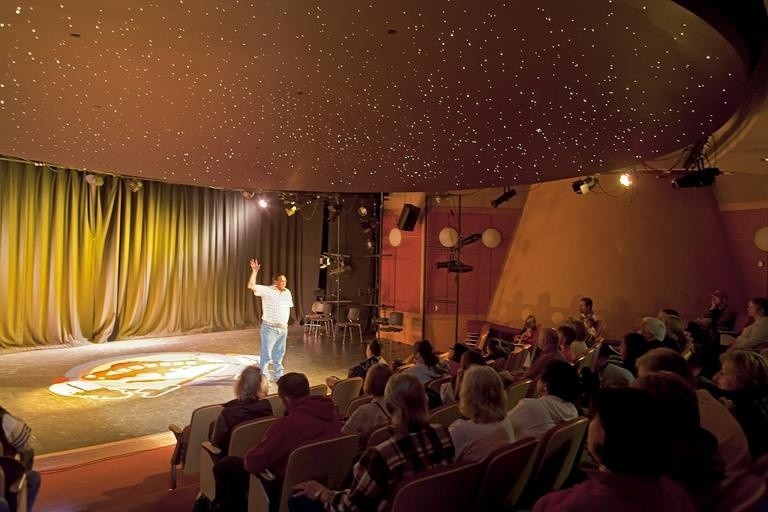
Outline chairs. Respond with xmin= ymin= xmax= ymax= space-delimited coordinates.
xmin=258 ymin=394 xmax=285 ymax=417
xmin=427 ymin=374 xmax=452 ymax=397
xmin=330 ymin=377 xmax=373 ymax=421
xmin=247 ymin=434 xmax=359 ymax=512
xmin=438 ymin=352 xmax=449 ymax=363
xmin=377 ymin=312 xmax=404 ymax=351
xmin=569 ymin=337 xmax=605 ymax=378
xmin=362 ymin=424 xmax=392 ymax=450
xmin=396 ymin=363 xmax=415 ymax=374
xmin=303 ymin=302 xmax=336 ymax=339
xmin=680 ymin=328 xmax=705 ymax=379
xmin=720 ymin=456 xmax=768 ymax=512
xmin=485 ymin=344 xmax=543 ymax=411
xmin=195 ymin=416 xmax=278 ymax=502
xmin=335 ymin=307 xmax=363 ymax=344
xmin=169 ymin=403 xmax=225 ymax=475
xmin=386 ymin=415 xmax=589 ymax=512
xmin=309 ymin=384 xmax=328 ymax=401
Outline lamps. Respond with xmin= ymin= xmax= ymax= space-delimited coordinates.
xmin=435 ymin=260 xmax=474 ymax=273
xmin=357 ymin=197 xmax=381 ymax=248
xmin=491 ymin=186 xmax=518 ymax=207
xmin=572 ymin=175 xmax=600 ymax=195
xmin=327 ymin=204 xmax=344 ymax=224
xmin=618 ymin=172 xmax=638 ymax=189
xmin=318 ymin=249 xmax=336 ymax=269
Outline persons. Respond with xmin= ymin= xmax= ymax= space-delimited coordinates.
xmin=246 ymin=258 xmax=300 ymax=385
xmin=0 ymin=407 xmax=40 ymax=511
